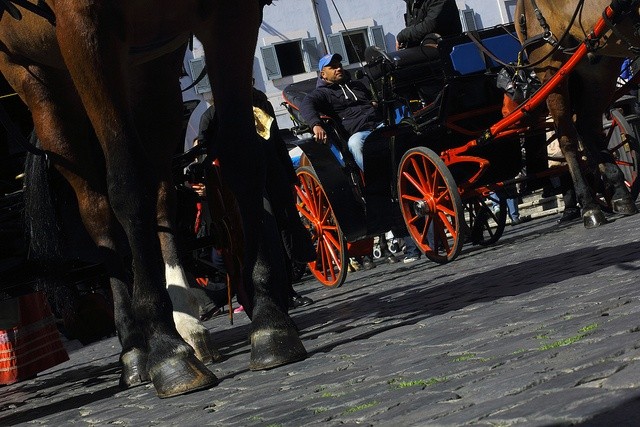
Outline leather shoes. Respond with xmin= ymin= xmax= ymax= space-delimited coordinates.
xmin=288 ymin=293 xmax=313 ymax=309
xmin=557 ymin=205 xmax=581 ymax=223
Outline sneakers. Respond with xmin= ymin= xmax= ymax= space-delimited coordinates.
xmin=234 ymin=305 xmax=246 ymax=313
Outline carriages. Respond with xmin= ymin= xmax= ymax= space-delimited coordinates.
xmin=281 ymin=0 xmax=638 ymax=288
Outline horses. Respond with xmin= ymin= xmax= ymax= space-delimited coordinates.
xmin=512 ymin=0 xmax=640 ymax=229
xmin=1 ymin=0 xmax=313 ymax=401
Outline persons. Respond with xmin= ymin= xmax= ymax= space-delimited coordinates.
xmin=490 ymin=191 xmax=520 ymax=225
xmin=299 ymin=52 xmax=388 ymax=173
xmin=401 ymin=235 xmax=423 ymax=263
xmin=184 ymin=84 xmax=318 ymax=322
xmin=395 ymin=0 xmax=462 ymax=106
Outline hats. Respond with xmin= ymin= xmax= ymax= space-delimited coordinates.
xmin=318 ymin=53 xmax=342 ymax=71
